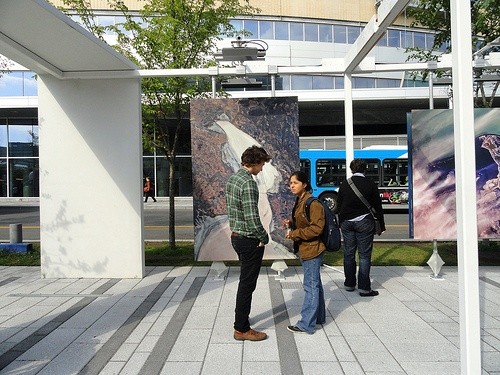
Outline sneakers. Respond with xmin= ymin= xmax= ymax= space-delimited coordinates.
xmin=234 ymin=329 xmax=268 ymax=341
xmin=287 ymin=325 xmax=306 ymax=333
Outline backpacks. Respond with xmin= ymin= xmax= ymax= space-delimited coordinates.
xmin=305 ymin=197 xmax=341 ymax=252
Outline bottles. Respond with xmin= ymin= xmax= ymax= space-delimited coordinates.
xmin=284 ymin=219 xmax=293 ymax=239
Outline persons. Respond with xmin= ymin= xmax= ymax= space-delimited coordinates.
xmin=400 ymin=176 xmax=408 ymax=186
xmin=337 ymin=158 xmax=387 ymax=297
xmin=282 ymin=171 xmax=326 ymax=334
xmin=388 ymin=176 xmax=398 ymax=186
xmin=226 ymin=146 xmax=273 ymax=341
xmin=144 ymin=177 xmax=157 ymax=203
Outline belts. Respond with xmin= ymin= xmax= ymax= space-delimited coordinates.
xmin=231 ymin=232 xmax=239 ymax=236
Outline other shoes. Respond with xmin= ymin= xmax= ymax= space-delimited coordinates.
xmin=359 ymin=291 xmax=378 ymax=296
xmin=346 ymin=289 xmax=354 ymax=291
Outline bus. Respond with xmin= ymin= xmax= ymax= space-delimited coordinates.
xmin=299 ymin=149 xmax=409 ymax=212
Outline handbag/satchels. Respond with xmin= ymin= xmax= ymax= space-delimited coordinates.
xmin=370 ymin=208 xmax=382 ymax=236
xmin=144 ymin=187 xmax=148 ymax=192
